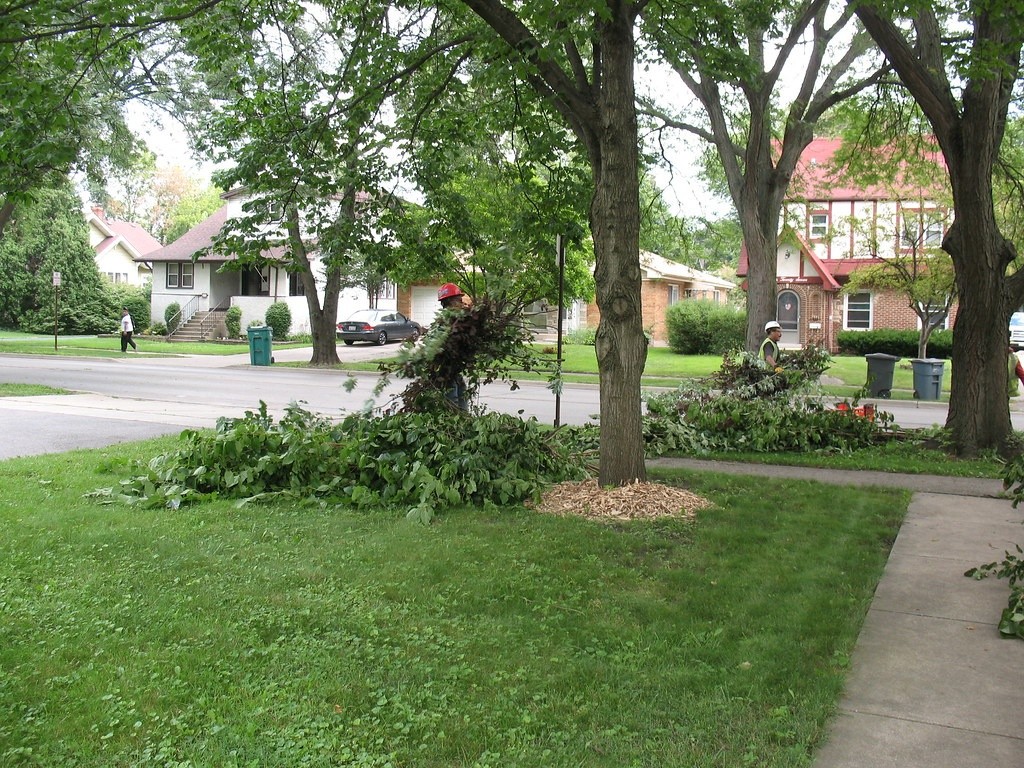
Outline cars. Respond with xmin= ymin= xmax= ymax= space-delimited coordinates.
xmin=335 ymin=309 xmax=423 ymax=348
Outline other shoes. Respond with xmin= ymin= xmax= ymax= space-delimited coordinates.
xmin=121 ymin=351 xmax=126 ymax=354
xmin=135 ymin=344 xmax=138 ymax=351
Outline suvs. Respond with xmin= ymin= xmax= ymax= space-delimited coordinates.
xmin=1008 ymin=312 xmax=1024 ymax=351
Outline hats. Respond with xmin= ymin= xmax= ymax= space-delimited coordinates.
xmin=122 ymin=306 xmax=127 ymax=310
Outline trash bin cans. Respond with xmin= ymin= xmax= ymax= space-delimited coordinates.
xmin=247 ymin=324 xmax=274 ymax=366
xmin=864 ymin=352 xmax=901 ymax=399
xmin=907 ymin=358 xmax=946 ymax=401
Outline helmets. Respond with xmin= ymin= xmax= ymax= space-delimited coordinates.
xmin=764 ymin=320 xmax=780 ymax=333
xmin=437 ymin=282 xmax=466 ymax=301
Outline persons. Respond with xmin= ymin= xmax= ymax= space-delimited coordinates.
xmin=758 ymin=318 xmax=788 ymax=402
xmin=1008 ymin=341 xmax=1024 ymax=399
xmin=120 ymin=307 xmax=139 ymax=353
xmin=437 ymin=283 xmax=471 ymax=413
